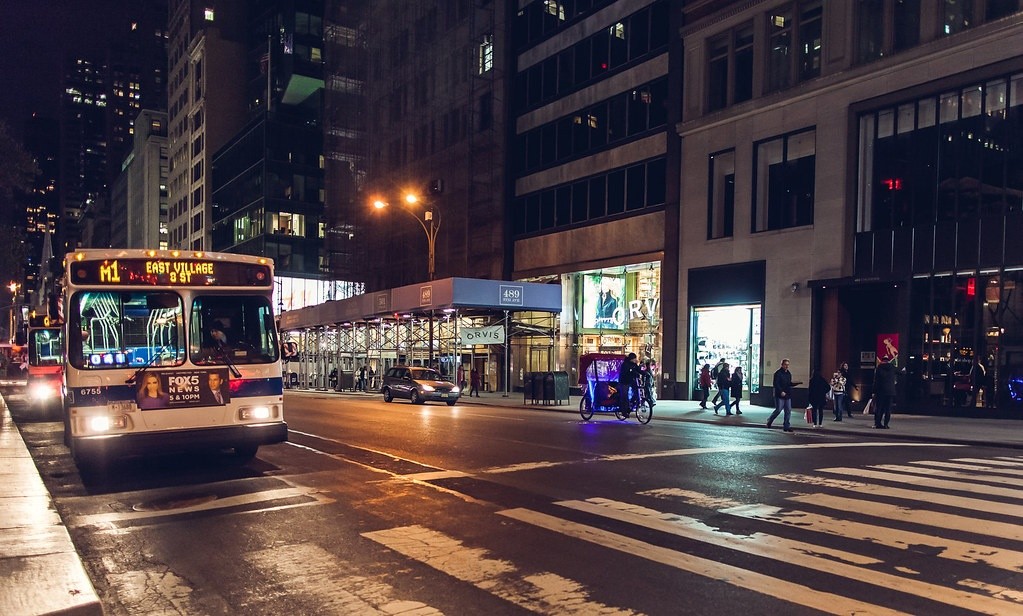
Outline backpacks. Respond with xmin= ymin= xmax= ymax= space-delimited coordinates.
xmin=711 ymin=362 xmax=722 ymax=380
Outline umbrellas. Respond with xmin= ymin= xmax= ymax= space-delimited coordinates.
xmin=0 ymin=343 xmax=11 ymax=354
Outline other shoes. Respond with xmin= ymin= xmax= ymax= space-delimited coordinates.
xmin=712 ymin=400 xmax=717 ymax=405
xmin=848 ymin=415 xmax=854 ymax=418
xmin=652 ymin=403 xmax=656 ymax=407
xmin=622 ymin=411 xmax=629 ymax=418
xmin=784 ymin=427 xmax=793 ymax=432
xmin=726 ymin=412 xmax=733 ymax=415
xmin=700 ymin=403 xmax=708 ymax=409
xmin=834 ymin=417 xmax=842 ymax=421
xmin=873 ymin=425 xmax=884 ymax=429
xmin=736 ymin=410 xmax=742 ymax=414
xmin=883 ymin=424 xmax=889 ymax=429
xmin=819 ymin=425 xmax=823 ymax=427
xmin=714 ymin=407 xmax=718 ymax=414
xmin=767 ymin=419 xmax=771 ymax=428
xmin=813 ymin=424 xmax=818 ymax=428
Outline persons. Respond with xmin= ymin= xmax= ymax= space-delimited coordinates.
xmin=595 ymin=278 xmax=620 ymax=329
xmin=619 ymin=353 xmax=656 ymax=418
xmin=332 ymin=368 xmax=338 ymax=389
xmin=828 ymin=361 xmax=859 ymax=421
xmin=139 ymin=372 xmax=170 ymax=408
xmin=872 ymin=355 xmax=898 ymax=429
xmin=967 ymin=359 xmax=986 ymax=407
xmin=711 ymin=358 xmax=725 ymax=406
xmin=81 ymin=331 xmax=91 ymax=350
xmin=729 ymin=367 xmax=743 ymax=414
xmin=700 ymin=364 xmax=713 ymax=409
xmin=355 ymin=366 xmax=374 ymax=391
xmin=202 ymin=371 xmax=229 ymax=405
xmin=767 ymin=358 xmax=803 ymax=432
xmin=808 ymin=368 xmax=831 ymax=427
xmin=210 ymin=320 xmax=241 ymax=347
xmin=457 ymin=365 xmax=465 ymax=397
xmin=470 ymin=368 xmax=480 ymax=397
xmin=714 ymin=363 xmax=733 ymax=415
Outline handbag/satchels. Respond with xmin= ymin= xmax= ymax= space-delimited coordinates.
xmin=806 ymin=405 xmax=813 ymax=423
xmin=863 ymin=398 xmax=876 ymax=414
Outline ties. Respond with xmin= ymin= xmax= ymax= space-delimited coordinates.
xmin=219 ymin=339 xmax=225 ymax=346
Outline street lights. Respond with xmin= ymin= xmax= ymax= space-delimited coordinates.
xmin=373 ymin=195 xmax=442 ymax=369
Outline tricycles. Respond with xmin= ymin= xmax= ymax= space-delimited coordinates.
xmin=578 ymin=352 xmax=652 ymax=425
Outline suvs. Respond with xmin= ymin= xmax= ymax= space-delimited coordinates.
xmin=382 ymin=364 xmax=460 ymax=406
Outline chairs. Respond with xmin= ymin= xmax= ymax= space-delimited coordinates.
xmin=121 ymin=345 xmax=184 ymax=366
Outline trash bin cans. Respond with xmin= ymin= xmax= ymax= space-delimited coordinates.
xmin=523 ymin=372 xmax=540 ymax=399
xmin=290 ymin=372 xmax=297 ymax=384
xmin=545 ymin=370 xmax=569 ymax=400
xmin=533 ymin=371 xmax=549 ymax=400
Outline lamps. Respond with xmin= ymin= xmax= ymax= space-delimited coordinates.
xmin=599 ymin=271 xmax=603 ymax=276
xmin=622 ymin=267 xmax=628 ymax=274
xmin=792 ymin=282 xmax=800 ymax=292
xmin=649 ymin=264 xmax=654 ymax=271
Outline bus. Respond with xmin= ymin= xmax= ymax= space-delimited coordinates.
xmin=24 ymin=325 xmax=64 ymax=405
xmin=60 ymin=247 xmax=298 ymax=471
